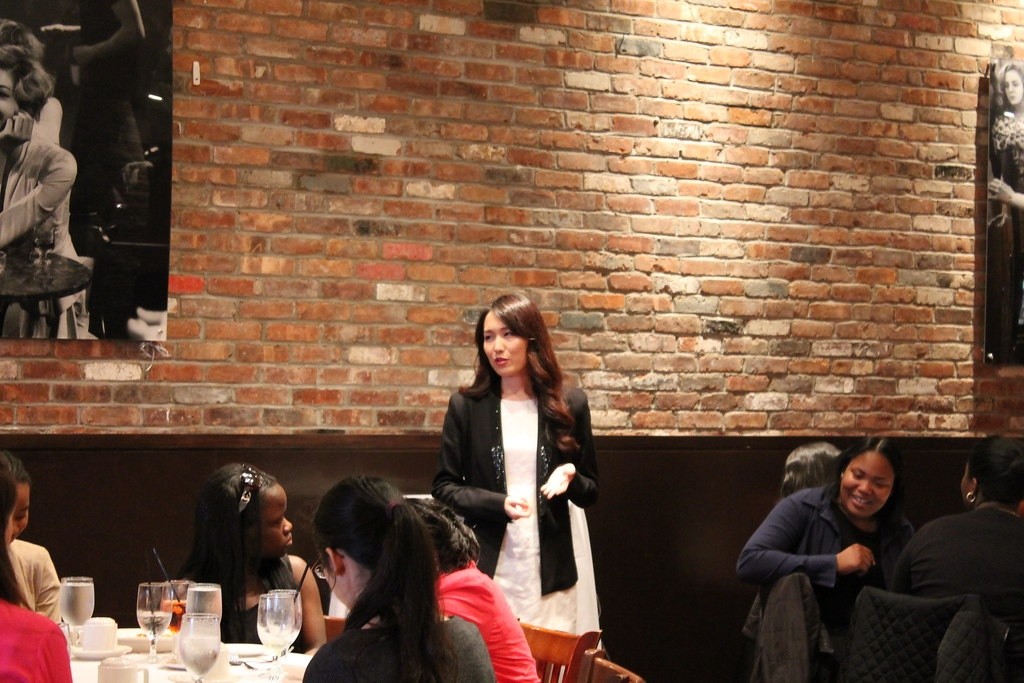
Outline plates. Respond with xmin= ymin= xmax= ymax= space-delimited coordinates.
xmin=69 ymin=646 xmax=132 ymax=660
xmin=116 ymin=639 xmax=173 ymax=652
xmin=225 ymin=644 xmax=263 ymax=657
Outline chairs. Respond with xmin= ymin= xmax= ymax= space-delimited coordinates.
xmin=516 ymin=616 xmax=603 ymax=683
xmin=577 ymin=649 xmax=646 ymax=683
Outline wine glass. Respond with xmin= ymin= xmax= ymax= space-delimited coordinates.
xmin=268 ymin=589 xmax=302 ymax=656
xmin=137 ymin=583 xmax=172 ymax=664
xmin=178 ymin=613 xmax=221 ymax=683
xmin=257 ymin=593 xmax=295 ymax=660
xmin=161 ymin=580 xmax=198 ymax=664
xmin=60 ymin=577 xmax=94 ymax=646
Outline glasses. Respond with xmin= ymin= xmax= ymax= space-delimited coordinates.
xmin=238 ymin=462 xmax=261 ymax=515
xmin=311 ymin=547 xmax=344 ymax=580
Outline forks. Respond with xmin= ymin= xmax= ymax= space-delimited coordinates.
xmin=269 ymin=675 xmax=279 ymax=681
xmin=229 ymin=648 xmax=242 ymax=665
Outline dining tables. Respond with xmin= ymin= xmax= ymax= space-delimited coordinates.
xmin=70 ymin=628 xmax=314 ymax=683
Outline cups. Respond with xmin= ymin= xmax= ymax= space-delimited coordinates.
xmin=186 ymin=583 xmax=222 ymax=623
xmin=57 ymin=623 xmax=71 ymax=657
xmin=98 ymin=657 xmax=149 ymax=683
xmin=85 ymin=617 xmax=118 ymax=650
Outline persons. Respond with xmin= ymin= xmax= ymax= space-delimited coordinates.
xmin=989 ymin=64 xmax=1024 ymax=254
xmin=300 ymin=473 xmax=496 ymax=683
xmin=179 ymin=462 xmax=327 ymax=656
xmin=889 ymin=436 xmax=1024 ymax=683
xmin=431 ymin=294 xmax=602 ymax=683
xmin=407 ymin=498 xmax=541 ymax=683
xmin=738 ymin=436 xmax=916 ymax=649
xmin=0 ymin=451 xmax=74 ymax=683
xmin=0 ymin=0 xmax=145 ymax=339
